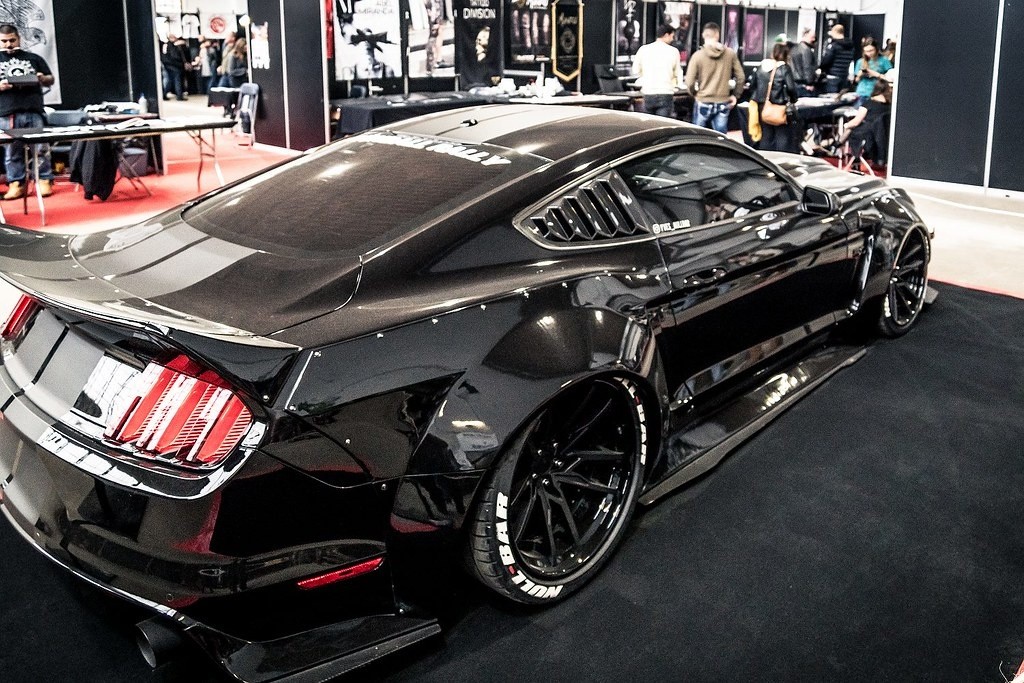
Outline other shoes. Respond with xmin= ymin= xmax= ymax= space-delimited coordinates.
xmin=161 ymin=92 xmax=170 ymax=101
xmin=4 ymin=180 xmax=25 ymax=200
xmin=850 ymin=163 xmax=862 ymax=173
xmin=175 ymin=96 xmax=187 ymax=101
xmin=835 ymin=136 xmax=842 ymax=148
xmin=37 ymin=178 xmax=52 ymax=197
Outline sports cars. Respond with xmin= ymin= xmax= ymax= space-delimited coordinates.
xmin=1 ymin=102 xmax=939 ymax=683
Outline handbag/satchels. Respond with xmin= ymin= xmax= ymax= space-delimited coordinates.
xmin=761 ymin=100 xmax=788 ymax=125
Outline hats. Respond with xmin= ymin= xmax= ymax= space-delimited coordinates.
xmin=774 ymin=32 xmax=791 ymax=43
xmin=830 ymin=25 xmax=845 ymax=40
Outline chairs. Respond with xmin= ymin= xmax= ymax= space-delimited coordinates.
xmin=227 ymin=83 xmax=260 ymax=150
xmin=840 ymin=112 xmax=891 ymax=177
xmin=90 ymin=116 xmax=154 ymax=197
xmin=44 ymin=111 xmax=86 ymax=154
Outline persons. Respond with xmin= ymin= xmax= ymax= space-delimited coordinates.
xmin=410 ymin=433 xmax=467 ymax=517
xmin=704 ymin=195 xmax=733 ymax=225
xmin=156 ymin=18 xmax=896 ymax=173
xmin=0 ymin=24 xmax=56 ymax=201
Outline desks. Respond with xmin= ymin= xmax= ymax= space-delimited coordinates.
xmin=3 ymin=112 xmax=238 ymax=230
xmin=332 ymin=88 xmax=696 ymax=153
